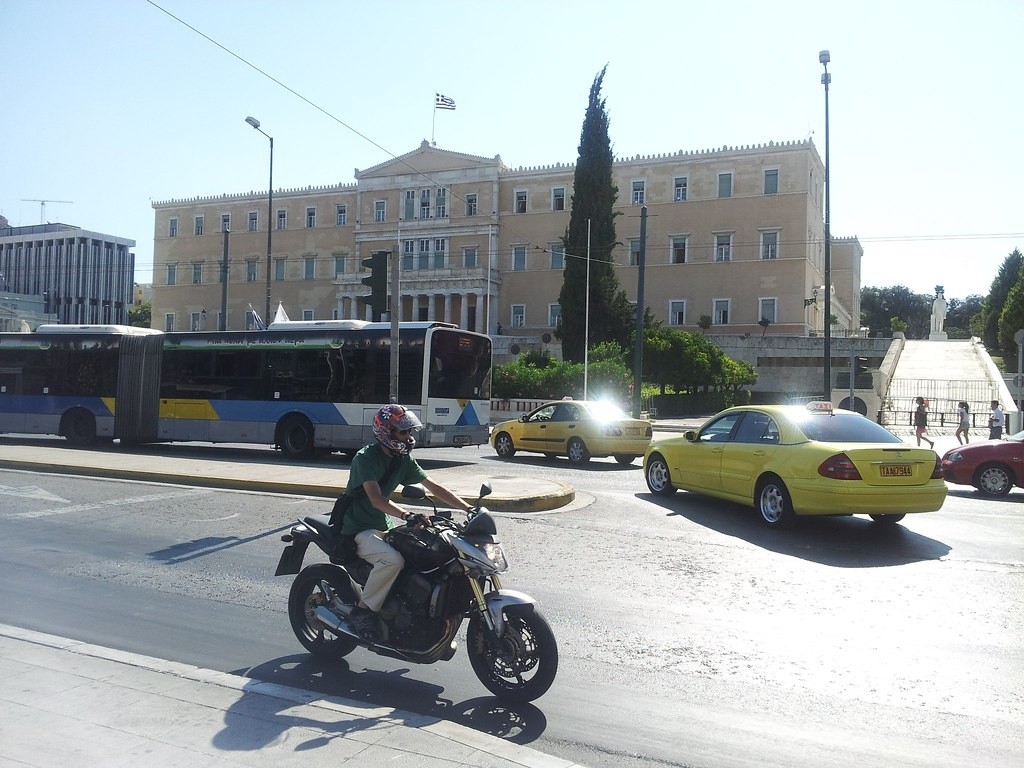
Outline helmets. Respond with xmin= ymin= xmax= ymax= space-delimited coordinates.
xmin=372 ymin=404 xmax=422 ymax=455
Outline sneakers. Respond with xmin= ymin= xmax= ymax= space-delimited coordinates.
xmin=350 ymin=606 xmax=378 ymax=643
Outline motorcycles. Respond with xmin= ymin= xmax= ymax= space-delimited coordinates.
xmin=273 ymin=481 xmax=559 ymax=701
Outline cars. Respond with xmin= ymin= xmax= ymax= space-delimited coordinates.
xmin=941 ymin=429 xmax=1024 ymax=498
xmin=489 ymin=395 xmax=653 ymax=467
xmin=643 ymin=400 xmax=949 ymax=533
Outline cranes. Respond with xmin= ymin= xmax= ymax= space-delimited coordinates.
xmin=21 ymin=199 xmax=73 ymax=225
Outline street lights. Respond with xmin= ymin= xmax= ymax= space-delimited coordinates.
xmin=245 ymin=115 xmax=274 ymax=331
xmin=818 ymin=48 xmax=833 ymax=399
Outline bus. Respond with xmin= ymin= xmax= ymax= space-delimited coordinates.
xmin=0 ymin=320 xmax=493 ymax=459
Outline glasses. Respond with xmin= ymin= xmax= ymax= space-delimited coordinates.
xmin=393 ymin=428 xmax=411 ymax=435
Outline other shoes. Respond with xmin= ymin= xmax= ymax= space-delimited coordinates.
xmin=930 ymin=441 xmax=934 ymax=449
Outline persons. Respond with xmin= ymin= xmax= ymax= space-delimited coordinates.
xmin=336 ymin=405 xmax=478 ymax=644
xmin=537 ymin=415 xmax=553 ymax=421
xmin=913 ymin=397 xmax=934 ymax=449
xmin=989 ymin=401 xmax=1004 ymax=441
xmin=956 ymin=402 xmax=971 ymax=445
xmin=933 ymin=293 xmax=947 ymax=331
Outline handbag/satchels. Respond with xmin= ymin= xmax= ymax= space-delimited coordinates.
xmin=989 ymin=419 xmax=993 ymax=429
xmin=915 ymin=406 xmax=928 ymax=426
xmin=328 ymin=491 xmax=353 ymax=529
